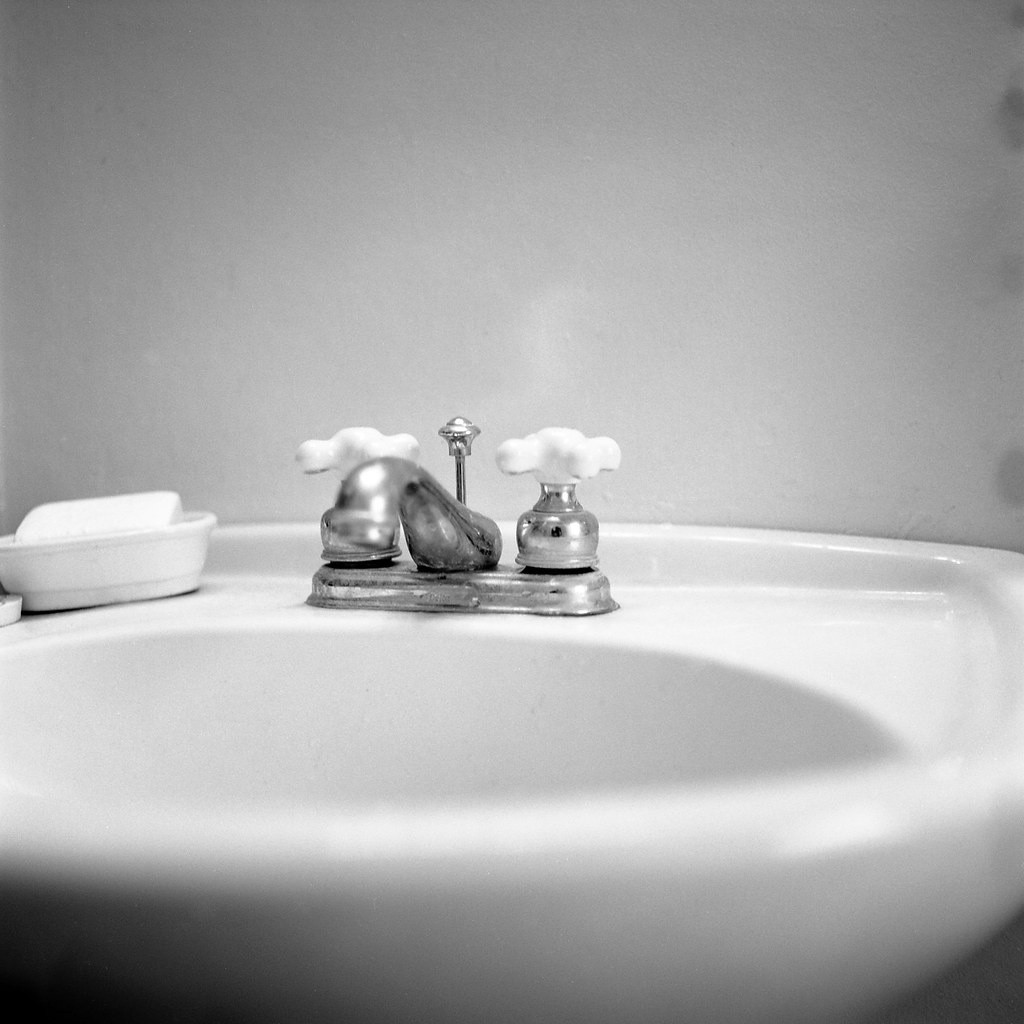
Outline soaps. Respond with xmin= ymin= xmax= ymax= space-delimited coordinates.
xmin=12 ymin=486 xmax=184 ymax=548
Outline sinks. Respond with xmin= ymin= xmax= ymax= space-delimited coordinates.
xmin=0 ymin=523 xmax=1023 ymax=1024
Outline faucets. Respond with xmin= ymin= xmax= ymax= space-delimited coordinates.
xmin=320 ymin=457 xmax=505 ymax=575
